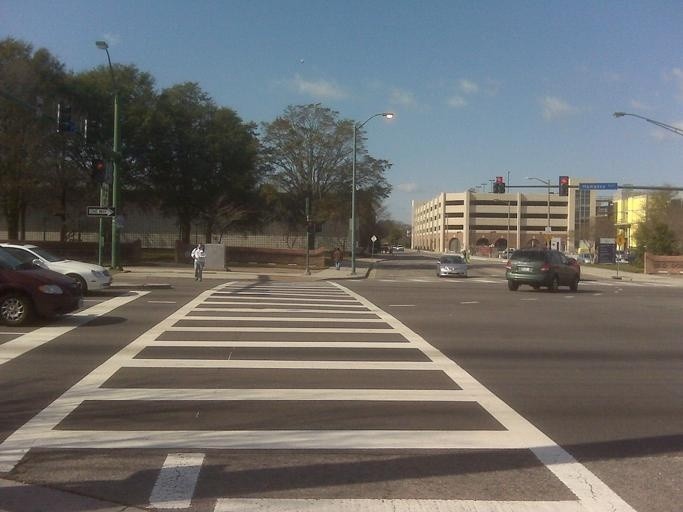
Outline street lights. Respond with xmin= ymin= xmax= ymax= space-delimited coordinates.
xmin=526 ymin=176 xmax=551 ymax=249
xmin=489 ymin=180 xmax=495 ymax=192
xmin=481 ymin=183 xmax=487 ymax=192
xmin=611 ymin=110 xmax=682 ymax=139
xmin=96 ymin=40 xmax=122 ymax=269
xmin=351 ymin=111 xmax=395 ymax=273
xmin=476 ymin=186 xmax=482 ymax=193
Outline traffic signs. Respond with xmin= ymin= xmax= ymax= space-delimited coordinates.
xmin=87 ymin=205 xmax=116 ymax=218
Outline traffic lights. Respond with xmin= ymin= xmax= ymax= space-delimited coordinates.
xmin=495 ymin=176 xmax=503 ymax=184
xmin=558 ymin=175 xmax=568 ymax=196
xmin=55 ymin=102 xmax=72 ymax=134
xmin=83 ymin=118 xmax=98 ymax=145
xmin=95 ymin=159 xmax=105 ymax=185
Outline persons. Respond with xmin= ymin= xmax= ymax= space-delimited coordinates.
xmin=333 ymin=248 xmax=343 ymax=270
xmin=191 ymin=243 xmax=207 ymax=282
xmin=462 ymin=251 xmax=468 ymax=263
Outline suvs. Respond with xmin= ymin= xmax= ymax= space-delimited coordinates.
xmin=505 ymin=247 xmax=582 ymax=294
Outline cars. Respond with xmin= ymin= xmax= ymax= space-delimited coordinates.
xmin=0 ymin=246 xmax=83 ymax=329
xmin=436 ymin=253 xmax=469 ymax=279
xmin=392 ymin=244 xmax=396 ymax=250
xmin=397 ymin=244 xmax=404 ymax=251
xmin=1 ymin=244 xmax=114 ymax=295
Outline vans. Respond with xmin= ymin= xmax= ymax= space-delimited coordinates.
xmin=580 ymin=252 xmax=591 ymax=264
